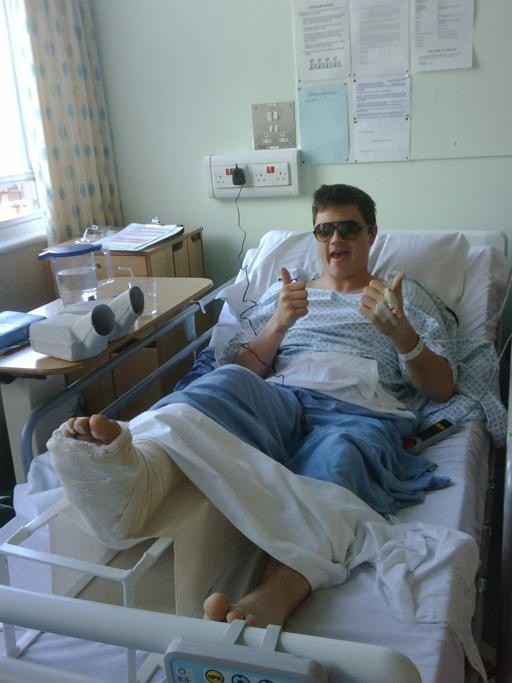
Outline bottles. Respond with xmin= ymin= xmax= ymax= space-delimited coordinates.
xmin=152 ymin=216 xmax=160 ymax=225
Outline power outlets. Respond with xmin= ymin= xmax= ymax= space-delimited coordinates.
xmin=210 ymin=163 xmax=290 ymax=190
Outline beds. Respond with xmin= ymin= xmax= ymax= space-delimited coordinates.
xmin=0 ymin=229 xmax=508 ymax=683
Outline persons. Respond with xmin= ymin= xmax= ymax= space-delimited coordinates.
xmin=46 ymin=184 xmax=458 ymax=629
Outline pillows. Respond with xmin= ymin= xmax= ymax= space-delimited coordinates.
xmin=220 ymin=229 xmax=470 ymax=322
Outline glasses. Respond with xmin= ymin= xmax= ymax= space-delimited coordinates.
xmin=313 ymin=220 xmax=372 ymax=242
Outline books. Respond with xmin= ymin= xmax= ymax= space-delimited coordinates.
xmin=0 ymin=311 xmax=47 ymax=351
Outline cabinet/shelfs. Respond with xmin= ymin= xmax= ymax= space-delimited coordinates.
xmin=41 ymin=223 xmax=214 ymax=422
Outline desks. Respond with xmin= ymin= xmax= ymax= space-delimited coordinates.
xmin=0 ymin=275 xmax=215 ymax=483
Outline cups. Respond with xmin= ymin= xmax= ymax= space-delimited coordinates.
xmin=37 ymin=243 xmax=114 ymax=309
xmin=129 ymin=279 xmax=156 ymax=316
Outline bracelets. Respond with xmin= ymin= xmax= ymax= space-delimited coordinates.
xmin=398 ymin=333 xmax=425 ymax=361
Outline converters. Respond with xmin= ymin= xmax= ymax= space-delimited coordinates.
xmin=233 ymin=168 xmax=244 ymax=185
